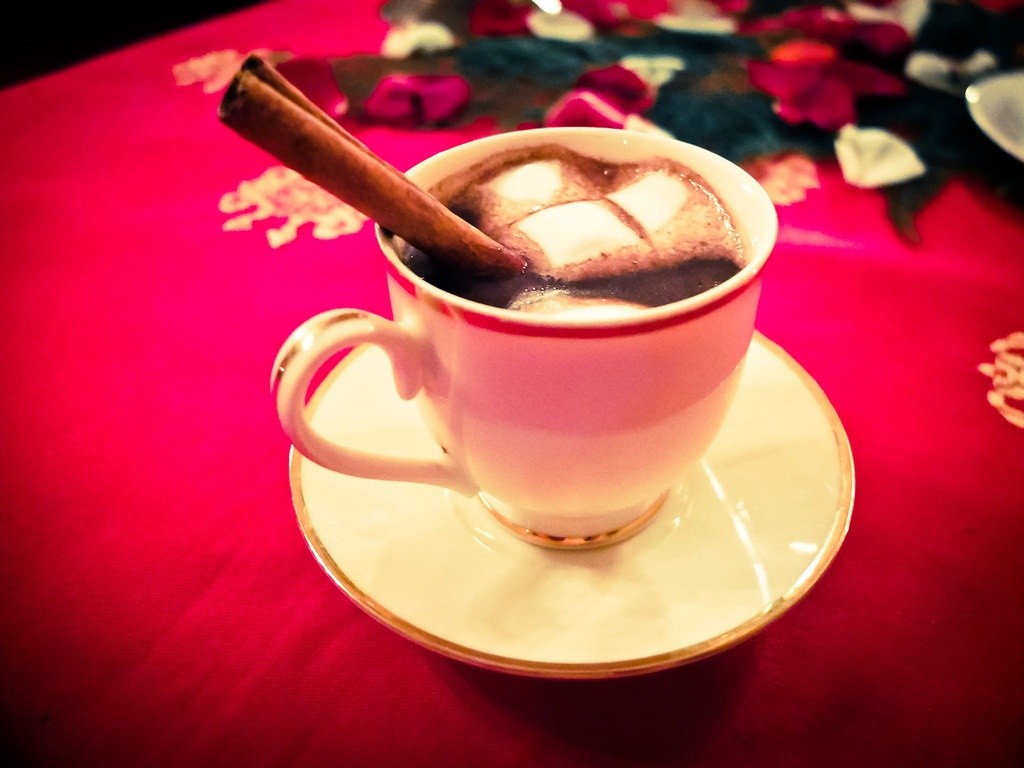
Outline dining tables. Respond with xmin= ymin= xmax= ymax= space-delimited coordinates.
xmin=0 ymin=0 xmax=1023 ymax=768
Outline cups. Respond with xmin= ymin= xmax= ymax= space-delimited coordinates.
xmin=270 ymin=127 xmax=779 ymax=550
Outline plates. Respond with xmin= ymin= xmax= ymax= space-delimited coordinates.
xmin=289 ymin=330 xmax=855 ymax=679
xmin=966 ymin=66 xmax=1024 ymax=162
